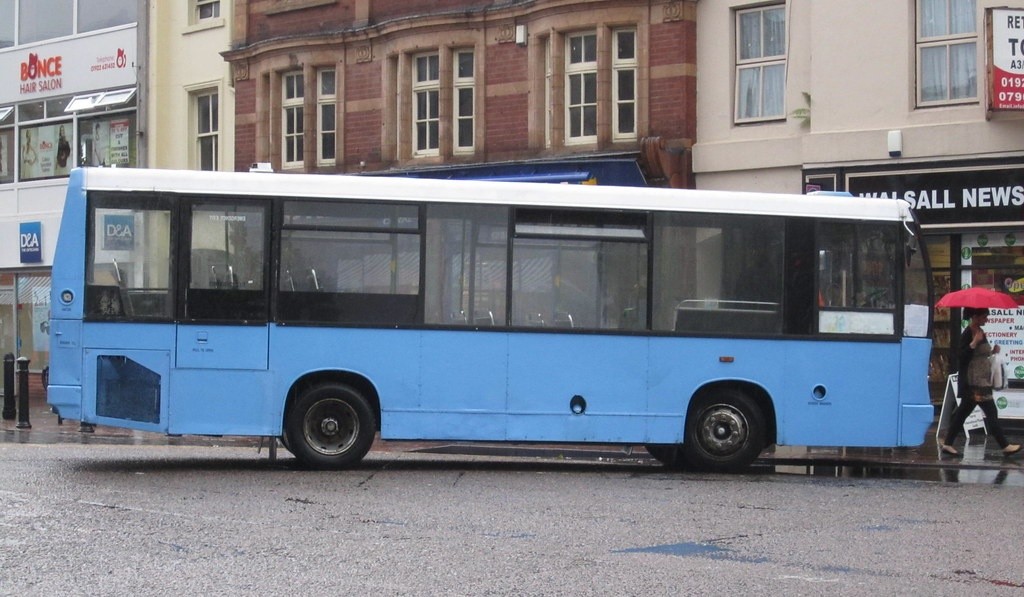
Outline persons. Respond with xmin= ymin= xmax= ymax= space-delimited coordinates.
xmin=941 ymin=307 xmax=1024 ymax=457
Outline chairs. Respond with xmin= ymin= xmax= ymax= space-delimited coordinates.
xmin=110 ymin=257 xmax=578 ymax=330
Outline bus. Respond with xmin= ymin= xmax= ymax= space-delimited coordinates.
xmin=45 ymin=167 xmax=938 ymax=474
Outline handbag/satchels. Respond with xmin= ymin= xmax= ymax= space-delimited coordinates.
xmin=987 ymin=352 xmax=1008 ymax=390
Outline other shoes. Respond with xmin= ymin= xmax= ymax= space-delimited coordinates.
xmin=942 ymin=446 xmax=963 ymax=458
xmin=1003 ymin=445 xmax=1024 ymax=455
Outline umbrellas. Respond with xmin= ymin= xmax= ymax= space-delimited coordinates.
xmin=934 ymin=287 xmax=1020 ymax=309
